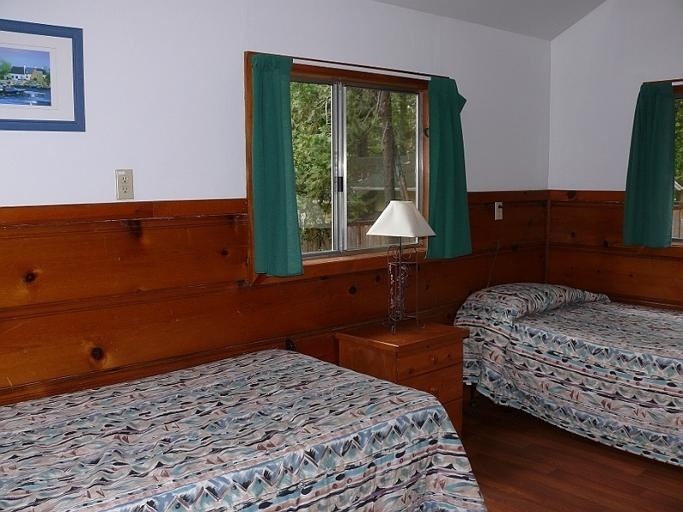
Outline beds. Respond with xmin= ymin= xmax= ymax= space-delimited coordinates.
xmin=469 ymin=304 xmax=683 ymax=474
xmin=0 ymin=349 xmax=440 ymax=510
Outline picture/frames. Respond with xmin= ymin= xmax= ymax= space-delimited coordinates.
xmin=0 ymin=15 xmax=89 ymax=133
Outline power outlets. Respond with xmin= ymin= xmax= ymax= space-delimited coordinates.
xmin=114 ymin=169 xmax=136 ymax=200
xmin=494 ymin=201 xmax=504 ymax=220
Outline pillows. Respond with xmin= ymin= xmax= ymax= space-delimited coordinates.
xmin=451 ymin=283 xmax=608 ymax=344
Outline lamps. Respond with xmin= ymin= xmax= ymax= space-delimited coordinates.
xmin=361 ymin=198 xmax=436 ymax=327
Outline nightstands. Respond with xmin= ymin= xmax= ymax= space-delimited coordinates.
xmin=339 ymin=319 xmax=469 ymax=448
